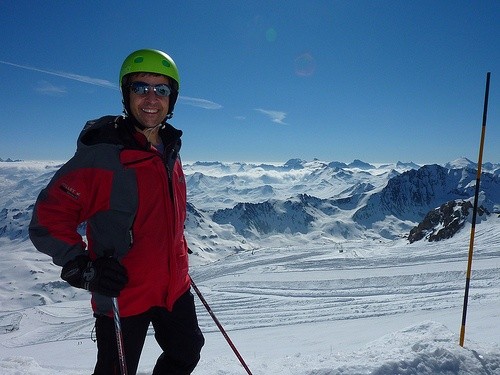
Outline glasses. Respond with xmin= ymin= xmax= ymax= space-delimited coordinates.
xmin=128 ymin=82 xmax=171 ymax=97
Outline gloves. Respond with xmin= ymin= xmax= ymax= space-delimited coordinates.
xmin=60 ymin=250 xmax=129 ymax=298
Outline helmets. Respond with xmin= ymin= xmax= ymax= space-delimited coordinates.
xmin=119 ymin=48 xmax=180 ymax=105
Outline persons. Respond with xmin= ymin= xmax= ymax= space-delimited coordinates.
xmin=28 ymin=48 xmax=205 ymax=375
